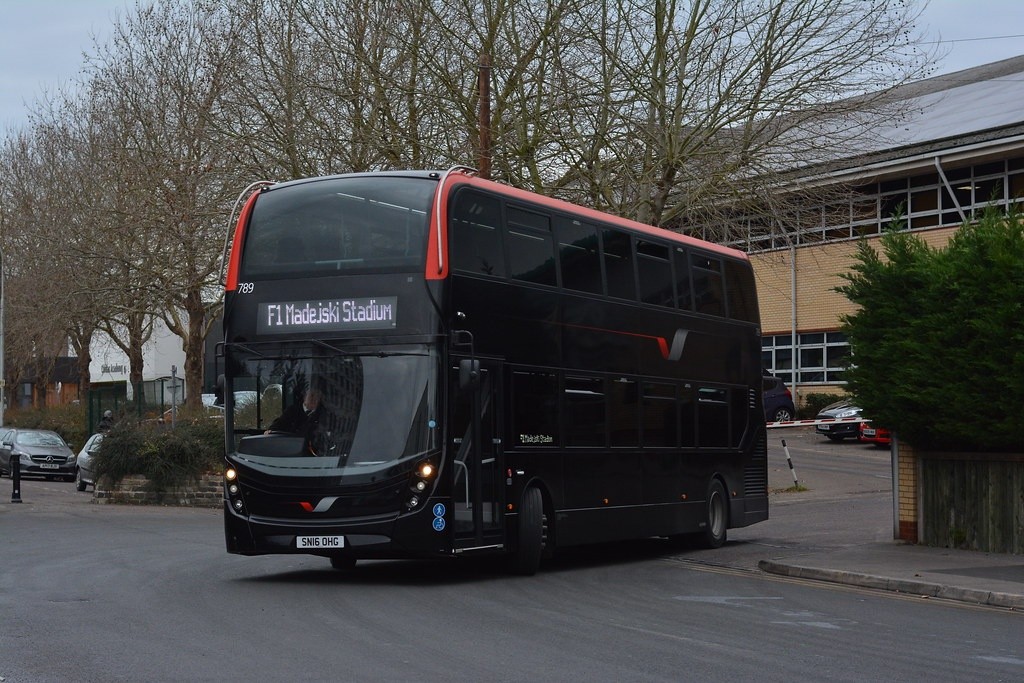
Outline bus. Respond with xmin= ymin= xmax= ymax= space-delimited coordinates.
xmin=208 ymin=165 xmax=769 ymax=576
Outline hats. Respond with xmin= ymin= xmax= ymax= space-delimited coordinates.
xmin=104 ymin=410 xmax=112 ymax=417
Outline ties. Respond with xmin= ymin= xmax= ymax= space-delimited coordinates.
xmin=305 ymin=410 xmax=312 ymax=415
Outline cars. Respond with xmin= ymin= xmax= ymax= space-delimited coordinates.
xmin=0 ymin=428 xmax=78 ymax=483
xmin=761 ymin=376 xmax=795 ymax=425
xmin=859 ymin=418 xmax=892 ymax=446
xmin=813 ymin=397 xmax=868 ymax=444
xmin=211 ymin=390 xmax=262 ymax=414
xmin=74 ymin=433 xmax=108 ymax=491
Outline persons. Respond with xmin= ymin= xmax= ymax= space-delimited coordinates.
xmin=96 ymin=410 xmax=116 ymax=432
xmin=264 ymin=384 xmax=336 ymax=457
